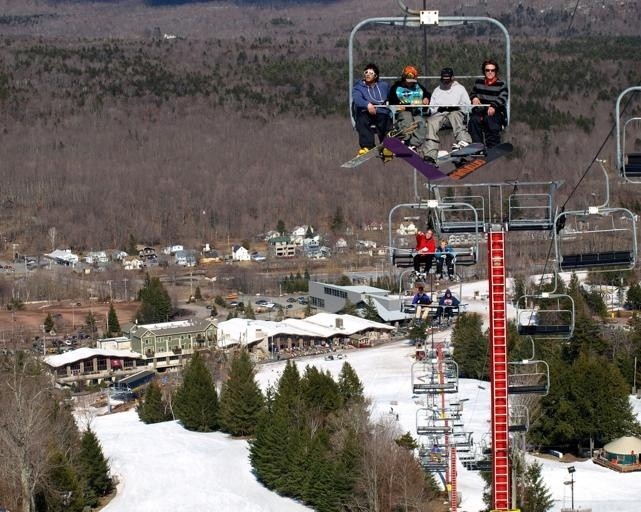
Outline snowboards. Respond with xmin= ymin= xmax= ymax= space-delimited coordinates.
xmin=414 ymin=281 xmax=425 ymax=288
xmin=340 ymin=119 xmax=513 ymax=184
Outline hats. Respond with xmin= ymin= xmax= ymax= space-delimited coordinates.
xmin=402 ymin=66 xmax=418 ymax=82
xmin=441 ymin=68 xmax=453 ymax=81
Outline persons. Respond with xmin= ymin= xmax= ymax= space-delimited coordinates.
xmin=434 ymin=240 xmax=456 ymax=281
xmin=435 ymin=289 xmax=460 ymax=325
xmin=423 ymin=67 xmax=471 ymax=164
xmin=387 ymin=66 xmax=431 ymax=152
xmin=467 ymin=59 xmax=508 ymax=157
xmin=412 ymin=286 xmax=430 ymax=325
xmin=413 ymin=230 xmax=436 ymax=278
xmin=351 ymin=62 xmax=392 ymax=158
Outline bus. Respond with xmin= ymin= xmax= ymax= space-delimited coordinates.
xmin=112 ymin=368 xmax=157 ymax=400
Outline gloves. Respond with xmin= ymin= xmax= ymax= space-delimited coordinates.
xmin=438 ymin=106 xmax=460 ymax=113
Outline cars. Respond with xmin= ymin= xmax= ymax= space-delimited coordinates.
xmin=31 ymin=332 xmax=92 ymax=354
xmin=206 ymin=293 xmax=308 ymax=312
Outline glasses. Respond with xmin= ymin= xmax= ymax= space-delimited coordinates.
xmin=485 ymin=68 xmax=496 ymax=72
xmin=364 ymin=70 xmax=375 ymax=76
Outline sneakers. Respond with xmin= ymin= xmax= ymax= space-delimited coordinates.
xmin=414 ymin=318 xmax=427 ymax=327
xmin=359 ymin=147 xmax=368 ymax=154
xmin=434 ymin=316 xmax=453 ymax=325
xmin=411 ymin=270 xmax=458 ymax=283
xmin=452 ymin=140 xmax=471 ymax=149
